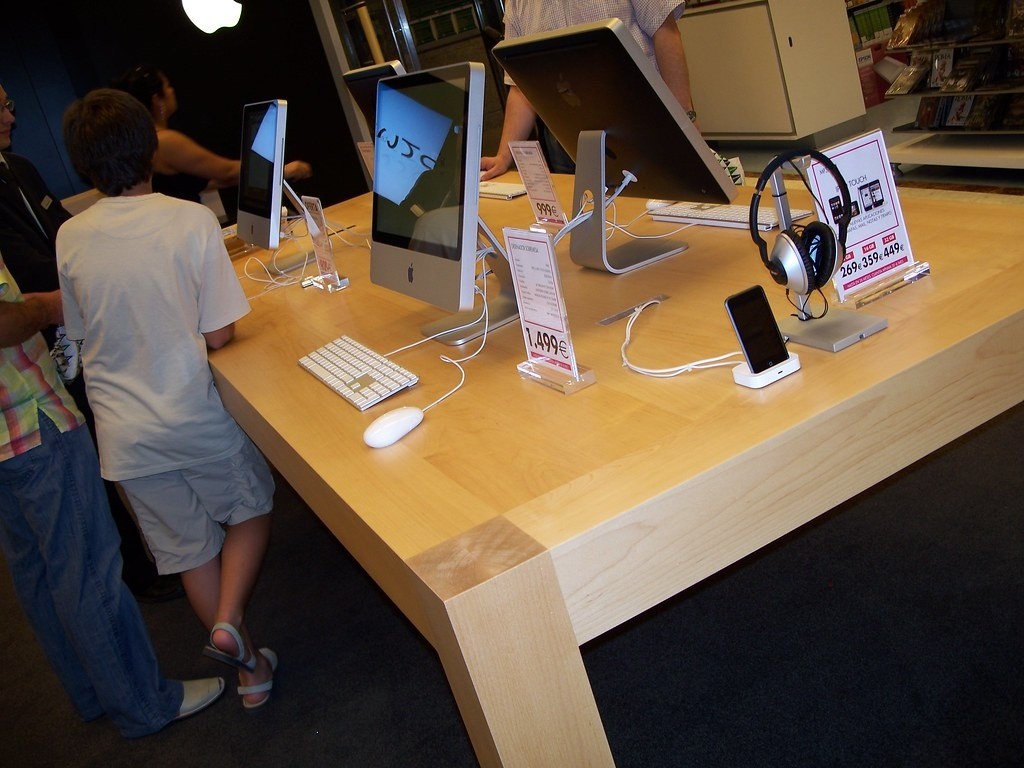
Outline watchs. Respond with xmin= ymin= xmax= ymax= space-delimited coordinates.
xmin=686 ymin=111 xmax=697 ymax=122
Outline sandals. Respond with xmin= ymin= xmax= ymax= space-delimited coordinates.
xmin=202 ymin=621 xmax=258 ymax=673
xmin=236 ymin=646 xmax=278 ymax=714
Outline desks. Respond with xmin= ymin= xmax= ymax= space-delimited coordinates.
xmin=207 ymin=162 xmax=1024 ymax=768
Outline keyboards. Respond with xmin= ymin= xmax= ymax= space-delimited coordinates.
xmin=296 ymin=336 xmax=420 ymax=411
xmin=479 ymin=181 xmax=527 ymax=201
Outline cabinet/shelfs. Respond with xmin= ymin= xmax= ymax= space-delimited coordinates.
xmin=886 ymin=37 xmax=1024 ymax=134
xmin=673 ymin=0 xmax=867 ymax=141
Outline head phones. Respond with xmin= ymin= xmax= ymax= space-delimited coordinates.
xmin=748 ymin=146 xmax=852 ymax=296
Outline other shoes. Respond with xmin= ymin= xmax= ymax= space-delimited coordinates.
xmin=170 ymin=676 xmax=226 ymax=720
xmin=127 ymin=560 xmax=185 ymax=602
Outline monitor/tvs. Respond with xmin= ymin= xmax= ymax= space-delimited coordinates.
xmin=237 ymin=18 xmax=739 ymax=347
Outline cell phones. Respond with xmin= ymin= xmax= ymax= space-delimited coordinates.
xmin=723 ymin=284 xmax=789 ymax=374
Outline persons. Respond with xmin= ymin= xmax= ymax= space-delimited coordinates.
xmin=124 ymin=65 xmax=313 ymax=204
xmin=0 ymin=85 xmax=77 ymax=351
xmin=54 ymin=84 xmax=282 ymax=711
xmin=477 ymin=0 xmax=701 ymax=182
xmin=0 ymin=218 xmax=228 ymax=737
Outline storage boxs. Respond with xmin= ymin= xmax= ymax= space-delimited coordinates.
xmin=847 ymin=0 xmax=910 ymax=107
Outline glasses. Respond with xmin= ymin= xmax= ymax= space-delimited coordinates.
xmin=0 ymin=99 xmax=15 ymax=113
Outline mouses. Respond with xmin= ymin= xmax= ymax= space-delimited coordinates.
xmin=363 ymin=407 xmax=423 ymax=448
xmin=479 ymin=170 xmax=489 ymax=178
